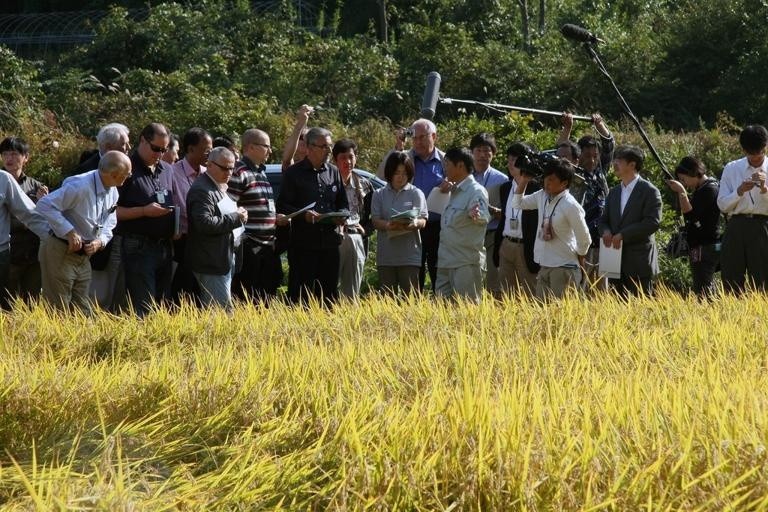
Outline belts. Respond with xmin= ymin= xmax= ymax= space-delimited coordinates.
xmin=505 ymin=235 xmax=525 ymax=244
xmin=732 ymin=213 xmax=768 ymax=221
xmin=555 ymin=264 xmax=578 ymax=271
xmin=344 ymin=226 xmax=362 ymax=236
xmin=48 ymin=228 xmax=71 ymax=246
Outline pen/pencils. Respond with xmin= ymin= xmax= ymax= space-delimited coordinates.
xmin=312 ymin=209 xmax=314 ymax=223
xmin=390 ymin=207 xmax=402 ymax=214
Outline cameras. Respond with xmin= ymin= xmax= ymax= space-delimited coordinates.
xmin=400 ymin=129 xmax=415 ymax=137
xmin=749 ymin=181 xmax=761 ymax=187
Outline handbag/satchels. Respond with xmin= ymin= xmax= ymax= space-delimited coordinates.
xmin=184 ymin=227 xmax=232 ymax=276
xmin=76 ymin=239 xmax=89 ymax=259
xmin=668 ymin=230 xmax=693 ymax=258
xmin=713 ymin=241 xmax=725 ymax=274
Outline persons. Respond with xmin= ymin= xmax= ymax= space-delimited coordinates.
xmin=35 ymin=150 xmax=132 ymax=319
xmin=332 ymin=138 xmax=376 ymax=304
xmin=0 ymin=169 xmax=50 ymax=314
xmin=0 ymin=136 xmax=49 ymax=313
xmin=187 ymin=147 xmax=248 ymax=319
xmin=594 ymin=146 xmax=662 ymax=305
xmin=374 ymin=151 xmax=428 ymax=307
xmin=281 ymin=104 xmax=314 ymax=171
xmin=513 ymin=151 xmax=592 ymax=309
xmin=494 ymin=143 xmax=540 ymax=305
xmin=469 ymin=134 xmax=509 ymax=299
xmin=226 ymin=128 xmax=288 ymax=316
xmin=665 ymin=156 xmax=721 ymax=304
xmin=377 ymin=119 xmax=457 ymax=301
xmin=161 ymin=134 xmax=180 ymax=316
xmin=211 ymin=137 xmax=246 ymax=307
xmin=555 ymin=141 xmax=587 ymax=215
xmin=127 ymin=123 xmax=175 ymax=320
xmin=717 ymin=125 xmax=768 ymax=300
xmin=556 ymin=111 xmax=615 ymax=298
xmin=70 ymin=123 xmax=131 ymax=314
xmin=173 ymin=127 xmax=213 ymax=312
xmin=276 ymin=127 xmax=347 ymax=312
xmin=435 ymin=146 xmax=489 ymax=305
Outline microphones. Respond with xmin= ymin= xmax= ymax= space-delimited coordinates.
xmin=560 ymin=25 xmax=606 ymax=46
xmin=420 ymin=72 xmax=441 ymax=118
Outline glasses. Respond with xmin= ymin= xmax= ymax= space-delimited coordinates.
xmin=210 ymin=161 xmax=234 ymax=170
xmin=413 ymin=133 xmax=430 ymax=141
xmin=313 ymin=144 xmax=334 ymax=151
xmin=255 ymin=144 xmax=271 ymax=148
xmin=143 ymin=134 xmax=170 ymax=153
xmin=748 ymin=151 xmax=766 ymax=157
xmin=612 ymin=159 xmax=623 ymax=166
xmin=122 ymin=173 xmax=133 ymax=178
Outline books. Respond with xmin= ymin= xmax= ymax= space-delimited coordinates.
xmin=169 ymin=205 xmax=180 ymax=235
xmin=315 ymin=212 xmax=349 ymax=226
